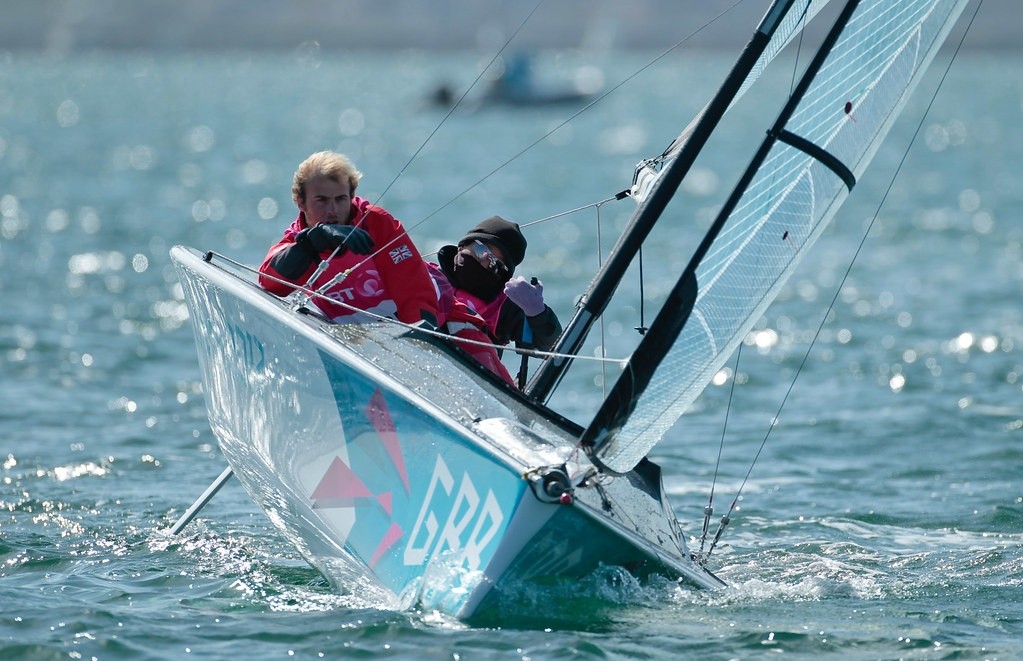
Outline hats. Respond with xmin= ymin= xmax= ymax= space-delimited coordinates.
xmin=455 ymin=215 xmax=528 ymax=283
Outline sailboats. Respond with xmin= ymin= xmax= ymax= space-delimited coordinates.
xmin=162 ymin=1 xmax=986 ymax=635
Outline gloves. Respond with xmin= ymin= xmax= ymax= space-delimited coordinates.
xmin=503 ymin=276 xmax=546 ymax=318
xmin=308 ymin=222 xmax=377 ymax=257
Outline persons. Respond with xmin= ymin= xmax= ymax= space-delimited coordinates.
xmin=259 ymin=150 xmax=519 ymax=390
xmin=436 ymin=215 xmax=563 ymax=359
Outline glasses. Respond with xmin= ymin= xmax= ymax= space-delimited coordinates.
xmin=465 ymin=238 xmax=511 ymax=284
